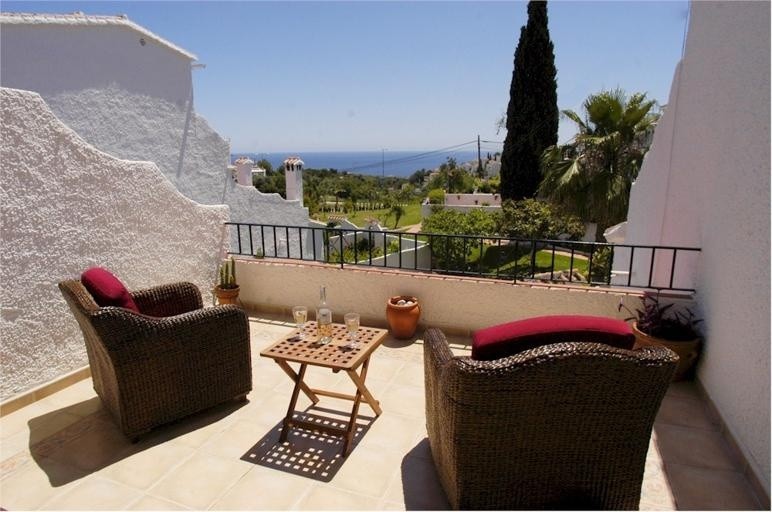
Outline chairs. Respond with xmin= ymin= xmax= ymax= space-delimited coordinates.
xmin=58 ymin=267 xmax=253 ymax=445
xmin=423 ymin=327 xmax=680 ymax=511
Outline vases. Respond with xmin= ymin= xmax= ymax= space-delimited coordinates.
xmin=386 ymin=296 xmax=421 ymax=340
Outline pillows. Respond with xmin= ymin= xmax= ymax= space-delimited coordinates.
xmin=81 ymin=268 xmax=140 ymax=313
xmin=471 ymin=315 xmax=635 ymax=361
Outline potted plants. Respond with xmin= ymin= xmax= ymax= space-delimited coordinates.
xmin=618 ymin=289 xmax=704 ymax=384
xmin=214 ymin=256 xmax=240 ymax=305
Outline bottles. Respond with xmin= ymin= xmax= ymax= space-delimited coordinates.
xmin=315 ymin=284 xmax=333 ymax=343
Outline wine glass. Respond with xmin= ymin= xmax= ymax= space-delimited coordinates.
xmin=345 ymin=313 xmax=360 ymax=347
xmin=293 ymin=306 xmax=307 ymax=338
